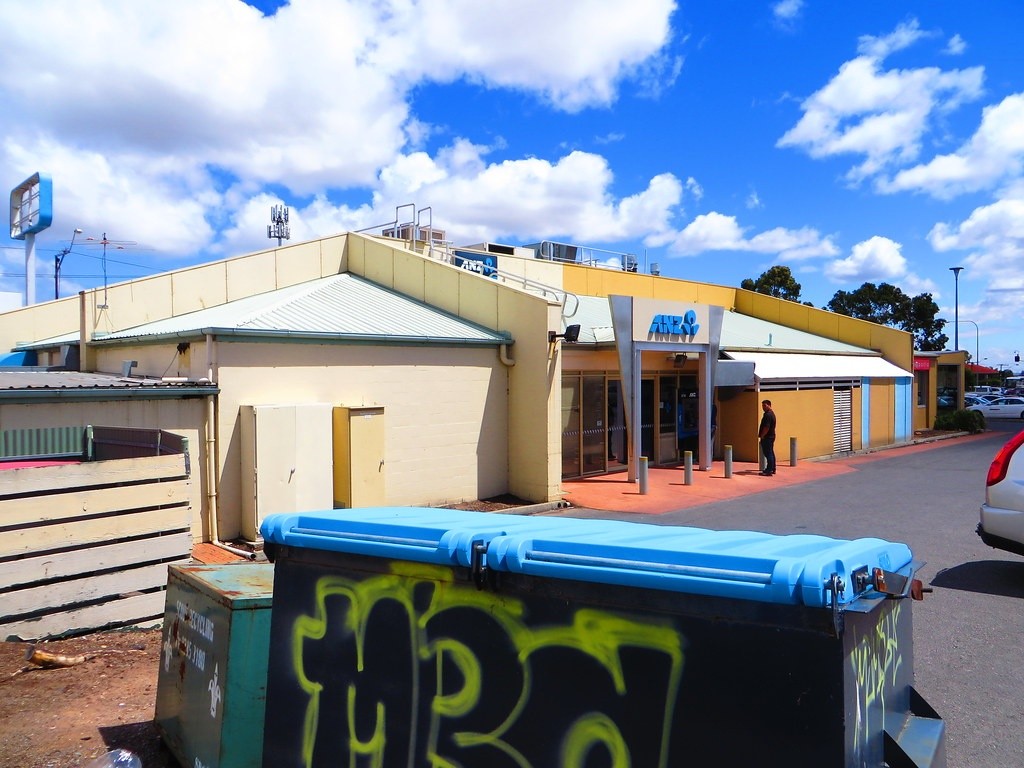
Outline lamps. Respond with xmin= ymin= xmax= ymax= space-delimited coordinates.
xmin=548 ymin=325 xmax=580 ymax=343
xmin=673 ymin=355 xmax=687 ymax=368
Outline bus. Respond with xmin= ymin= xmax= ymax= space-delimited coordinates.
xmin=1006 ymin=376 xmax=1024 ymax=389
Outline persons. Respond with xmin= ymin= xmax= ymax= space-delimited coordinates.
xmin=758 ymin=400 xmax=776 ymax=476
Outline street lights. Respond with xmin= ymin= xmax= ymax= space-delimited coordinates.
xmin=949 ymin=266 xmax=964 ymax=352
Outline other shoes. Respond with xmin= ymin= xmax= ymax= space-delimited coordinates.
xmin=758 ymin=469 xmax=776 ymax=476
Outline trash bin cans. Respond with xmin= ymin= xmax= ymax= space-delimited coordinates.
xmin=260 ymin=505 xmax=941 ymax=767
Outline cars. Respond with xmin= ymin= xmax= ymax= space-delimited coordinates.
xmin=965 ymin=397 xmax=1024 ymax=421
xmin=975 ymin=429 xmax=1024 ymax=557
xmin=936 ymin=386 xmax=1024 ymax=407
